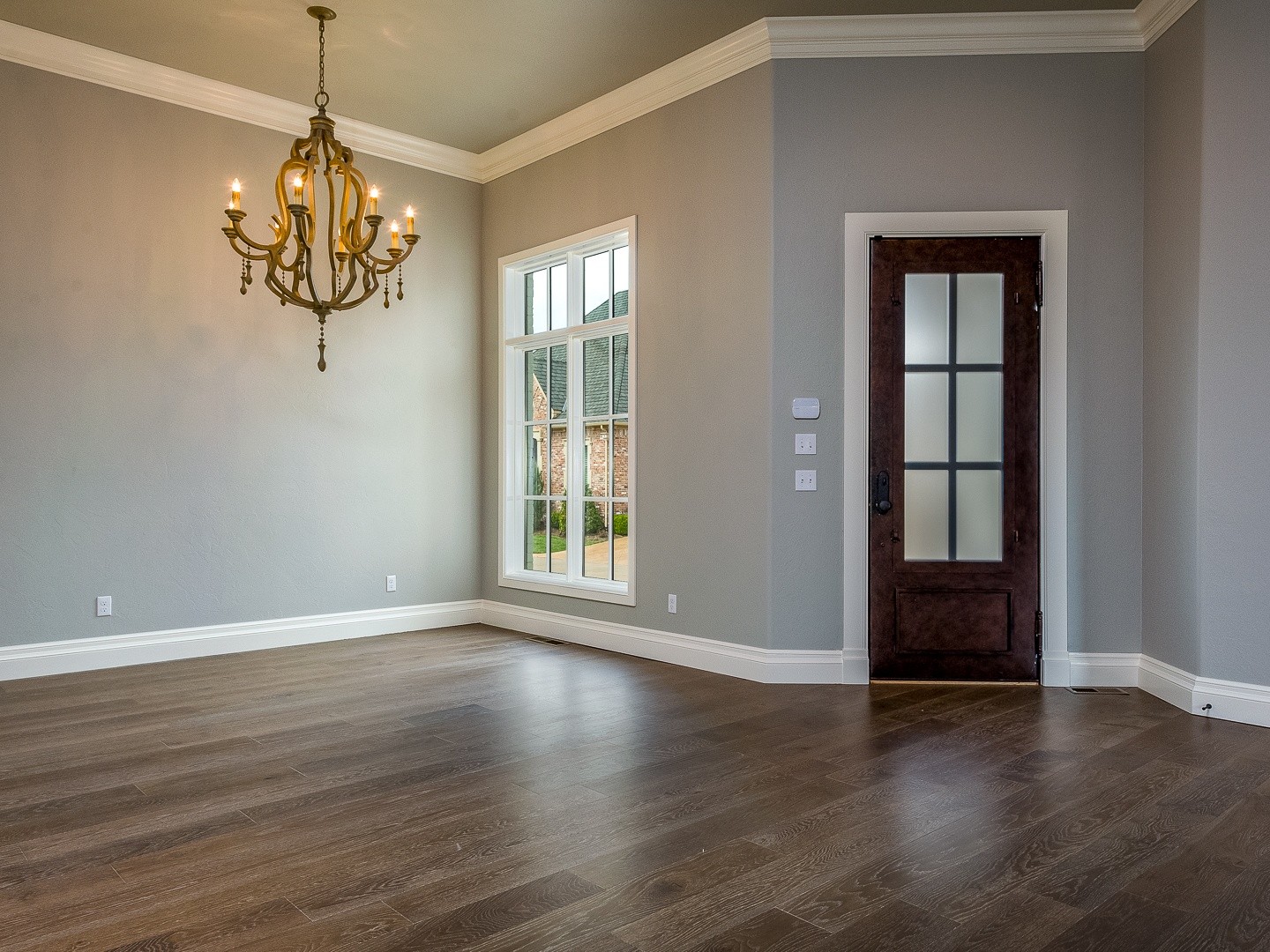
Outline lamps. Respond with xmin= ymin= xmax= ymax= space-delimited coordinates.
xmin=221 ymin=6 xmax=421 ymax=372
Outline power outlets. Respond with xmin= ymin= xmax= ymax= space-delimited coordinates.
xmin=97 ymin=596 xmax=111 ymax=616
xmin=668 ymin=594 xmax=677 ymax=613
xmin=387 ymin=575 xmax=396 ymax=592
xmin=795 ymin=433 xmax=816 ymax=454
xmin=795 ymin=470 xmax=817 ymax=491
